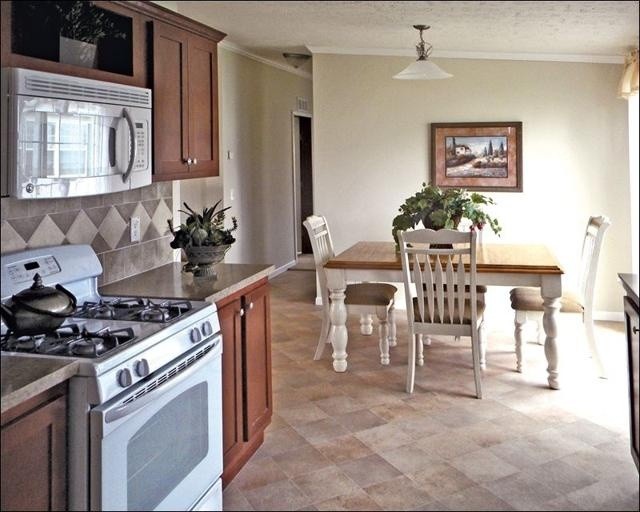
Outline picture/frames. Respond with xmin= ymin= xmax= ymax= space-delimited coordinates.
xmin=429 ymin=121 xmax=523 ymax=193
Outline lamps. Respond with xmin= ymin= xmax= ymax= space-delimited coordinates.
xmin=391 ymin=24 xmax=453 ymax=80
xmin=282 ymin=50 xmax=311 ymax=68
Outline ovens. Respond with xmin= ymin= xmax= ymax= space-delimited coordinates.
xmin=89 ymin=333 xmax=224 ymax=511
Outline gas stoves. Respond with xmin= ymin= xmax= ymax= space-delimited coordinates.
xmin=0 ymin=244 xmax=221 ymax=405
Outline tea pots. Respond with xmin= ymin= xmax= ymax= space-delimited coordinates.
xmin=0 ymin=272 xmax=77 ymax=336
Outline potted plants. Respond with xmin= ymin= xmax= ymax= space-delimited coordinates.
xmin=167 ymin=198 xmax=238 ymax=277
xmin=391 ymin=182 xmax=502 ymax=251
xmin=22 ymin=0 xmax=129 ymax=70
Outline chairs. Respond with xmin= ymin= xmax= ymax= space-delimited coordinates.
xmin=510 ymin=215 xmax=610 ymax=390
xmin=397 ymin=229 xmax=487 ymax=399
xmin=303 ymin=215 xmax=397 ymax=365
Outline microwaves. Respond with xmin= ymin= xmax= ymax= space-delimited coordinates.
xmin=1 ymin=67 xmax=152 ymax=200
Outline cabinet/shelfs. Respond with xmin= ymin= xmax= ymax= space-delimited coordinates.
xmin=98 ymin=261 xmax=277 ymax=491
xmin=142 ymin=0 xmax=227 ymax=182
xmin=617 ymin=273 xmax=640 ymax=472
xmin=0 ymin=356 xmax=81 ymax=512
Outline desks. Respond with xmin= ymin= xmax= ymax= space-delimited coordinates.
xmin=324 ymin=241 xmax=567 ymax=390
